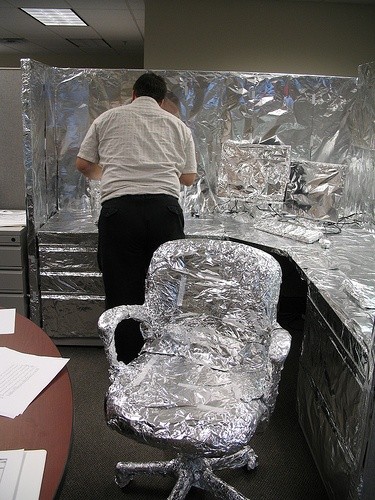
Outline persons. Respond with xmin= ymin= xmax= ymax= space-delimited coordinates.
xmin=77 ymin=73 xmax=196 ymax=365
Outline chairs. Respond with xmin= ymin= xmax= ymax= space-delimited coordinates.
xmin=97 ymin=238 xmax=292 ymax=500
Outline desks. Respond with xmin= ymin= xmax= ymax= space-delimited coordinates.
xmin=0 ymin=311 xmax=75 ymax=500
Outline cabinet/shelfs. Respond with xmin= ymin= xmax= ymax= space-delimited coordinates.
xmin=0 ymin=57 xmax=375 ymax=500
